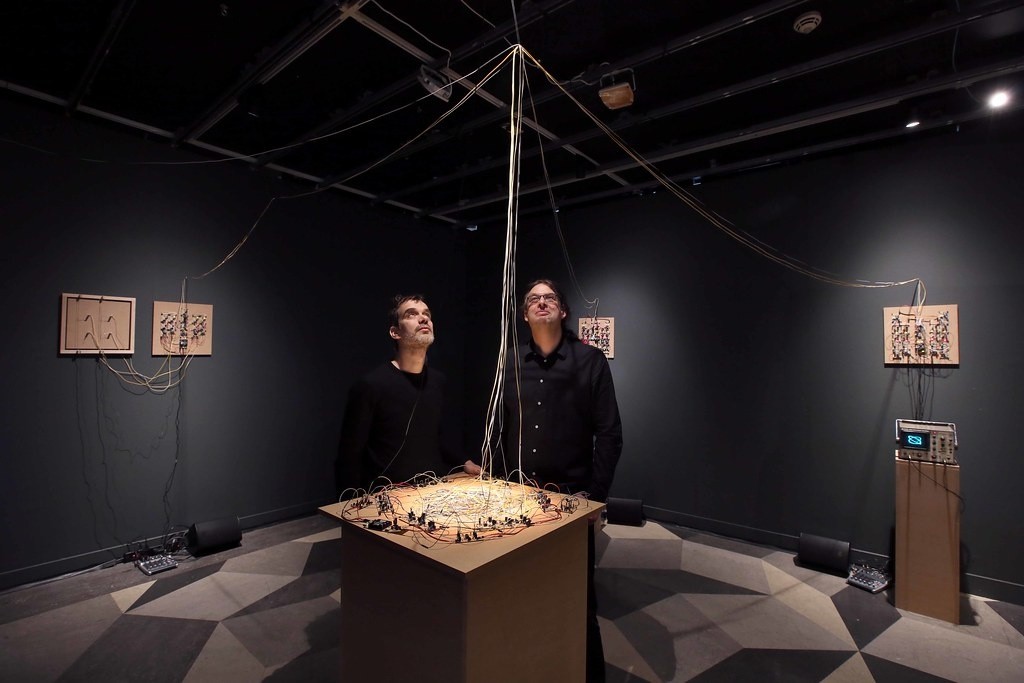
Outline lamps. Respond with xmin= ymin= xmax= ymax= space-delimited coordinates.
xmin=794 ymin=12 xmax=821 ymax=34
xmin=599 ymin=67 xmax=637 ymax=110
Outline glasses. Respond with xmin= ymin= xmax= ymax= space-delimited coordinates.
xmin=525 ymin=293 xmax=558 ymax=303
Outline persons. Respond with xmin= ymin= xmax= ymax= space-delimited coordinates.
xmin=489 ymin=279 xmax=625 ymax=683
xmin=350 ymin=293 xmax=491 ymax=499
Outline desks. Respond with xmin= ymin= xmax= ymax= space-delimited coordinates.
xmin=318 ymin=470 xmax=607 ymax=683
xmin=894 ymin=450 xmax=961 ymax=626
